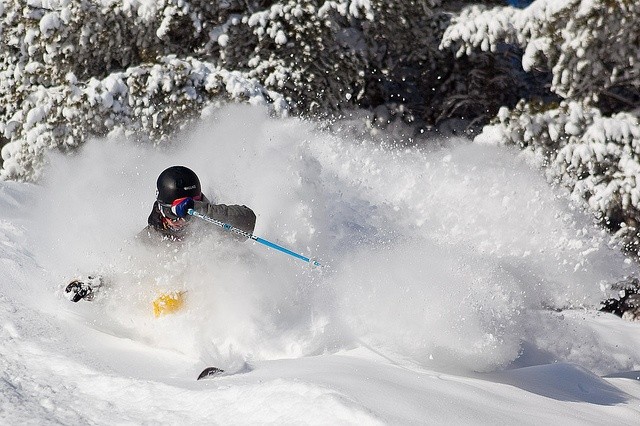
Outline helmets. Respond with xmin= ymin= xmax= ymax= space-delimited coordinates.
xmin=156 ymin=167 xmax=201 ymax=205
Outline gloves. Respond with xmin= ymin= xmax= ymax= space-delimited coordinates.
xmin=65 ymin=280 xmax=93 ymax=302
xmin=172 ymin=198 xmax=194 ymax=216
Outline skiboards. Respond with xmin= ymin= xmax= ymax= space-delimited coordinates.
xmin=195 ymin=361 xmax=252 ymax=380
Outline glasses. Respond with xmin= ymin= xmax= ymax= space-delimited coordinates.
xmin=157 ymin=202 xmax=195 ymax=222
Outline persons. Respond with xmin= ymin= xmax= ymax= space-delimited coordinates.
xmin=65 ymin=166 xmax=257 ymax=302
